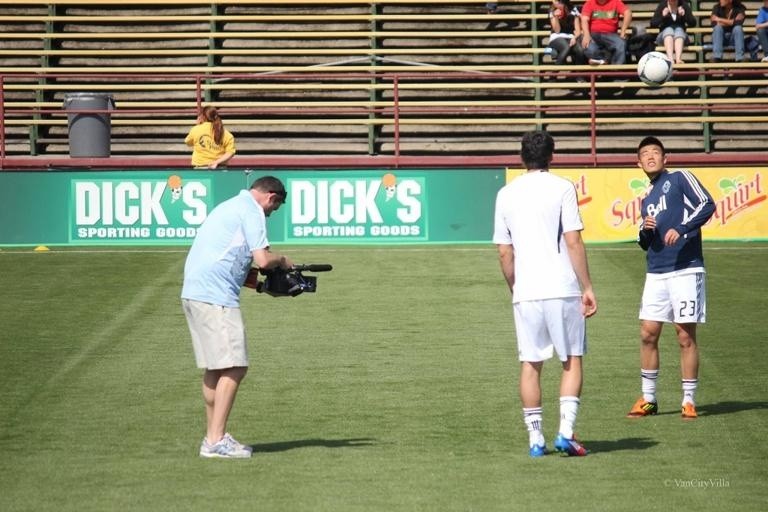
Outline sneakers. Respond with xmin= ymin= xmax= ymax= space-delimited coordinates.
xmin=680 ymin=399 xmax=697 ymax=418
xmin=627 ymin=397 xmax=659 ymax=419
xmin=199 ymin=436 xmax=251 ymax=459
xmin=528 ymin=440 xmax=549 ymax=457
xmin=224 ymin=431 xmax=254 ymax=452
xmin=553 ymin=432 xmax=589 ymax=457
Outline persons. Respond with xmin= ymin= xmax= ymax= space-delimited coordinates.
xmin=651 ymin=0 xmax=696 ymax=65
xmin=625 ymin=136 xmax=717 ymax=420
xmin=548 ymin=0 xmax=588 ymax=82
xmin=755 ymin=0 xmax=768 ymax=63
xmin=580 ymin=0 xmax=632 ymax=82
xmin=181 ymin=176 xmax=295 ymax=459
xmin=492 ymin=131 xmax=597 ymax=461
xmin=184 ymin=106 xmax=236 ymax=170
xmin=710 ymin=0 xmax=747 ymax=63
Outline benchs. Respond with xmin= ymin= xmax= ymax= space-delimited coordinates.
xmin=0 ymin=0 xmax=767 ymax=153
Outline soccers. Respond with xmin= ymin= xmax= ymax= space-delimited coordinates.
xmin=172 ymin=187 xmax=183 ymax=196
xmin=638 ymin=52 xmax=673 ymax=85
xmin=385 ymin=185 xmax=397 ymax=193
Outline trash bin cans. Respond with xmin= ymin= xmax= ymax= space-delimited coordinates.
xmin=62 ymin=92 xmax=117 ymax=158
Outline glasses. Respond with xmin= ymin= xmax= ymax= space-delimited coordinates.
xmin=269 ymin=189 xmax=288 ymax=198
